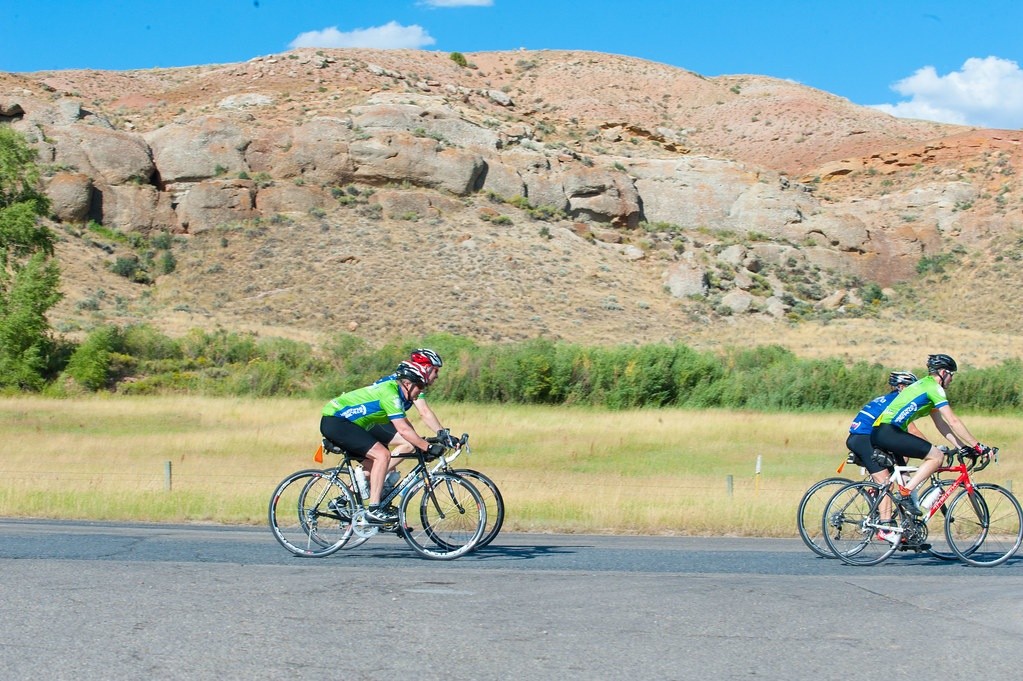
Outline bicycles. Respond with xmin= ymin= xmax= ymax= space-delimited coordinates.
xmin=267 ymin=442 xmax=487 ymax=562
xmin=822 ymin=446 xmax=1022 ymax=566
xmin=297 ymin=432 xmax=504 ymax=554
xmin=797 ymin=442 xmax=991 ymax=561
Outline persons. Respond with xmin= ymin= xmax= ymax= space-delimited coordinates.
xmin=319 ymin=360 xmax=444 ymax=526
xmin=844 ymin=369 xmax=949 ymax=546
xmin=363 ymin=349 xmax=462 ymax=537
xmin=871 ymin=352 xmax=995 ymax=552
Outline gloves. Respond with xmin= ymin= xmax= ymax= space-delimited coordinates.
xmin=428 ymin=444 xmax=447 ymax=458
xmin=421 ymin=450 xmax=437 ymax=463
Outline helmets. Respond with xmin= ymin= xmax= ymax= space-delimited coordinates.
xmin=888 ymin=371 xmax=919 ymax=387
xmin=410 ymin=348 xmax=443 ymax=367
xmin=925 ymin=354 xmax=957 ymax=373
xmin=395 ymin=360 xmax=429 ymax=385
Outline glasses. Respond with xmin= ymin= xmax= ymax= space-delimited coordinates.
xmin=402 ymin=375 xmax=425 ymax=390
xmin=942 ymin=368 xmax=954 ymax=377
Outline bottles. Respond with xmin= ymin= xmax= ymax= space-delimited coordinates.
xmin=380 ymin=470 xmax=401 ymax=497
xmin=354 ymin=466 xmax=370 ymax=500
xmin=906 ymin=480 xmax=920 ymax=509
xmin=921 ymin=487 xmax=945 ymax=511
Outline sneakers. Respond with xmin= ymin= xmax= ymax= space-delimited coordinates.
xmin=892 ymin=489 xmax=923 ymax=516
xmin=876 ymin=528 xmax=908 ymax=546
xmin=328 ymin=496 xmax=352 ymax=520
xmin=364 ymin=508 xmax=400 ymax=523
